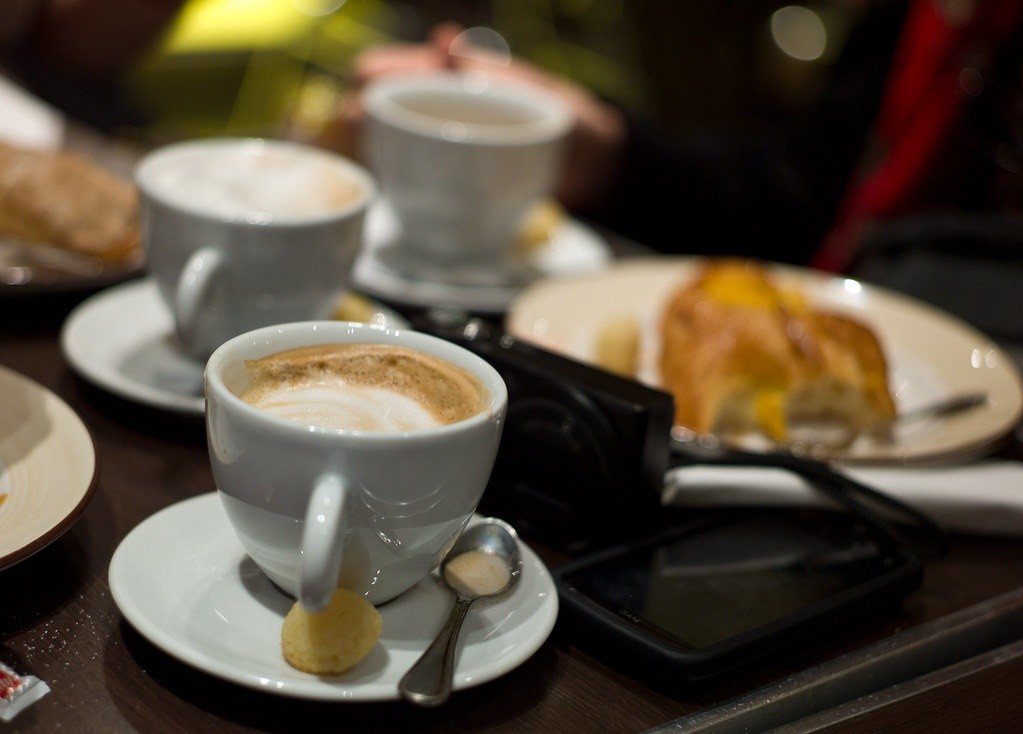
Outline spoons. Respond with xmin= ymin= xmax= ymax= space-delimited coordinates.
xmin=398 ymin=518 xmax=523 ymax=707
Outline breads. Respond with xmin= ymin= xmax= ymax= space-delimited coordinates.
xmin=281 ymin=587 xmax=382 ymax=674
xmin=0 ymin=142 xmax=145 ymax=281
xmin=664 ymin=266 xmax=900 ymax=442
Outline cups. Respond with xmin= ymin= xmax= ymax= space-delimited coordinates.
xmin=356 ymin=72 xmax=576 ymax=263
xmin=132 ymin=135 xmax=377 ymax=361
xmin=202 ymin=318 xmax=508 ymax=606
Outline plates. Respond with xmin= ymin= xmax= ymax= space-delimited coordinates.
xmin=109 ymin=490 xmax=561 ymax=701
xmin=351 ymin=199 xmax=614 ymax=312
xmin=0 ymin=361 xmax=101 ymax=571
xmin=504 ymin=253 xmax=1023 ymax=465
xmin=60 ymin=276 xmax=412 ymax=413
